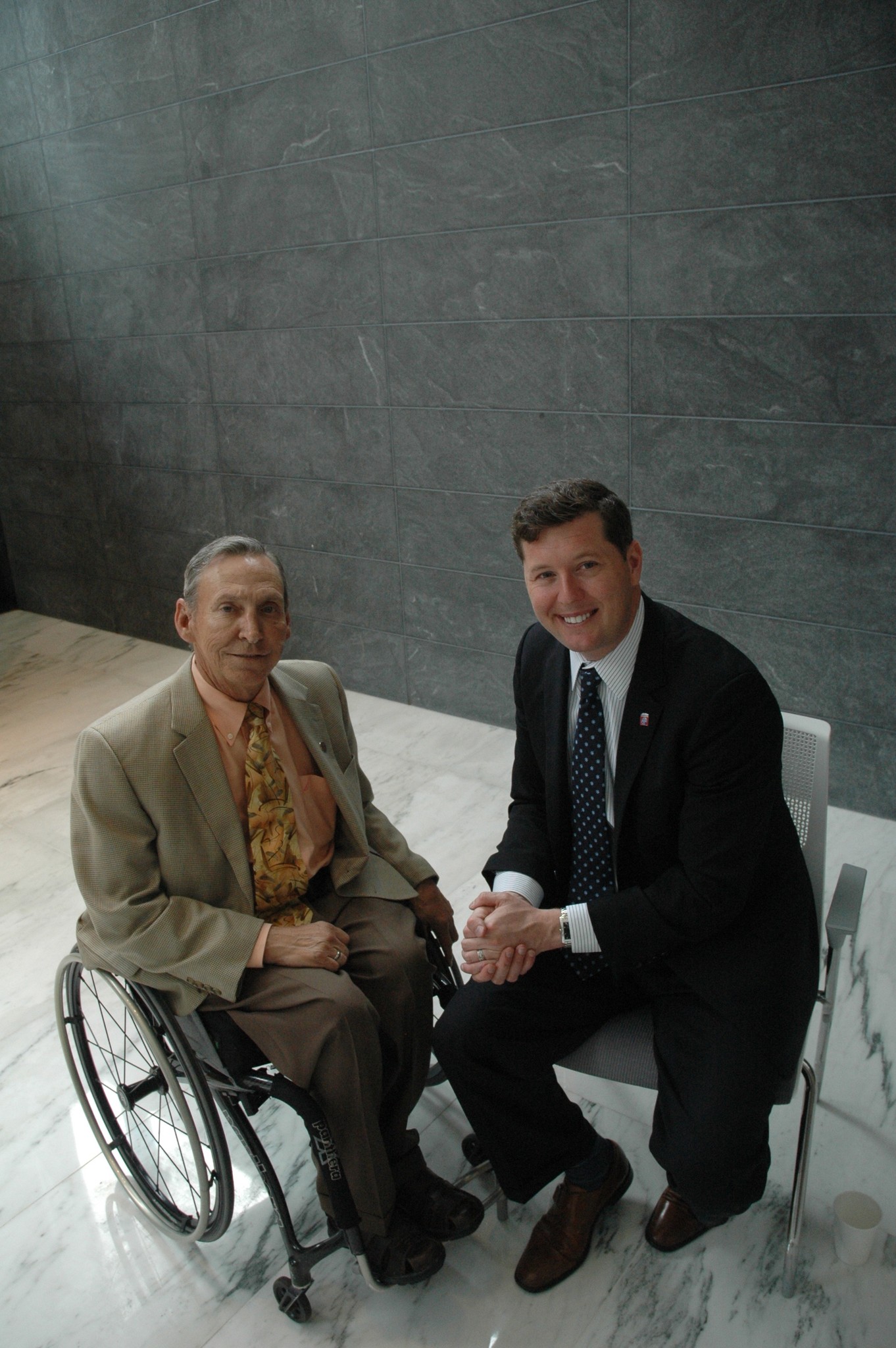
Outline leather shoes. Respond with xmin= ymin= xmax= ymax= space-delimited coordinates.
xmin=645 ymin=1184 xmax=727 ymax=1253
xmin=514 ymin=1135 xmax=633 ymax=1293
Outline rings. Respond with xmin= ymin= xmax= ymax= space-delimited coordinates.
xmin=333 ymin=950 xmax=341 ymax=960
xmin=477 ymin=950 xmax=485 ymax=961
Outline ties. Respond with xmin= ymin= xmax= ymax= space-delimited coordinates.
xmin=242 ymin=702 xmax=313 ymax=927
xmin=565 ymin=667 xmax=614 ymax=980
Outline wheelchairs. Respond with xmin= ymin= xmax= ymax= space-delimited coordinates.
xmin=53 ymin=899 xmax=525 ymax=1324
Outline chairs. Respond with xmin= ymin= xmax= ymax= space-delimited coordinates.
xmin=496 ymin=708 xmax=866 ymax=1300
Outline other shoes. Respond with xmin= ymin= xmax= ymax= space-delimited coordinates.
xmin=390 ymin=1163 xmax=484 ymax=1241
xmin=325 ymin=1209 xmax=445 ymax=1284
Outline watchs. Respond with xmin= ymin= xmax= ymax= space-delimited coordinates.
xmin=558 ymin=907 xmax=572 ymax=950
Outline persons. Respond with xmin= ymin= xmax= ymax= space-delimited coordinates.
xmin=430 ymin=481 xmax=820 ymax=1298
xmin=67 ymin=536 xmax=482 ymax=1289
